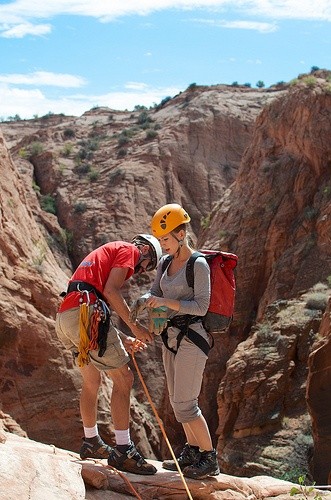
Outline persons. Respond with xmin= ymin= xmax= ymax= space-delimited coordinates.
xmin=128 ymin=204 xmax=220 ymax=480
xmin=55 ymin=233 xmax=162 ymax=475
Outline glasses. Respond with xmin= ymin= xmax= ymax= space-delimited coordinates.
xmin=146 ymin=250 xmax=154 ymax=273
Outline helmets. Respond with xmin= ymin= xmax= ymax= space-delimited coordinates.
xmin=152 ymin=204 xmax=192 ymax=237
xmin=132 ymin=233 xmax=162 ymax=273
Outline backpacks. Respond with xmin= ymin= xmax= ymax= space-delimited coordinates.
xmin=161 ymin=249 xmax=238 ymax=334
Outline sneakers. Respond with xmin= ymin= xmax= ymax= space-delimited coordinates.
xmin=163 ymin=444 xmax=200 ymax=470
xmin=108 ymin=441 xmax=158 ymax=476
xmin=182 ymin=447 xmax=220 ymax=479
xmin=80 ymin=436 xmax=111 ymax=459
